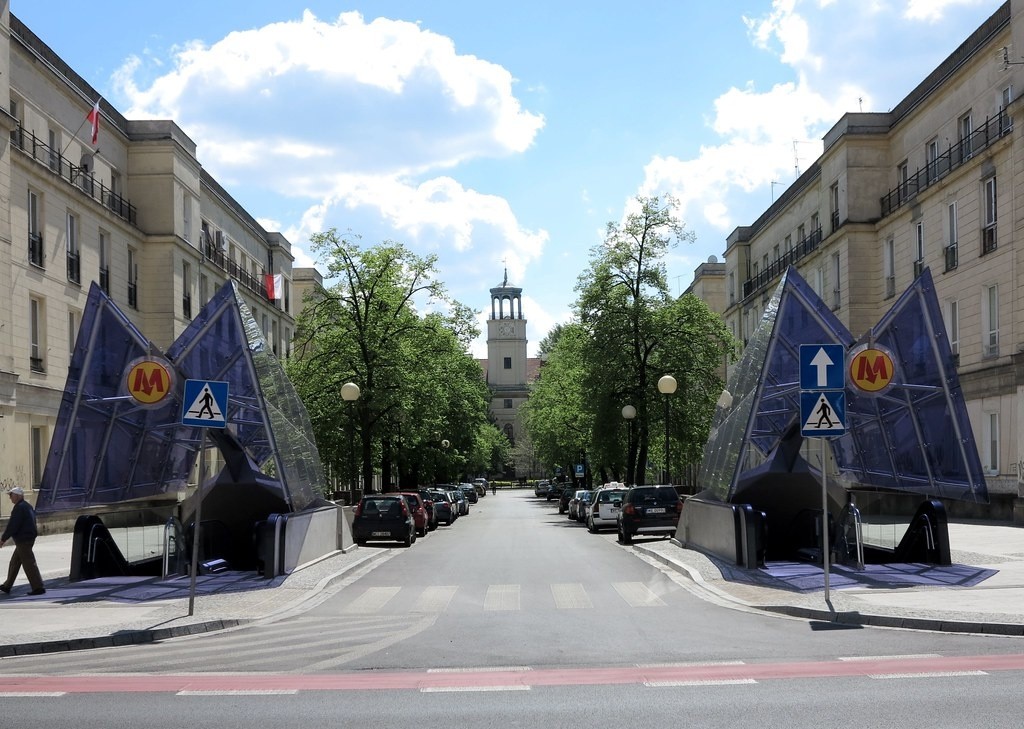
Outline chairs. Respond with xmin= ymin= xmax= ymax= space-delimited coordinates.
xmin=366 ymin=502 xmax=380 ymax=515
xmin=659 ymin=491 xmax=669 ymax=503
xmin=602 ymin=495 xmax=610 ymax=501
xmin=634 ymin=492 xmax=645 ymax=505
xmin=386 ymin=502 xmax=400 ymax=516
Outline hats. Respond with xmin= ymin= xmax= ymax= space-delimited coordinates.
xmin=7 ymin=487 xmax=24 ymax=495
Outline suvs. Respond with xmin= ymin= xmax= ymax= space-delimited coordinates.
xmin=352 ymin=493 xmax=417 ymax=547
xmin=616 ymin=478 xmax=684 ymax=544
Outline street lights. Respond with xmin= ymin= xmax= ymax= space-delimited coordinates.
xmin=658 ymin=375 xmax=677 ymax=483
xmin=341 ymin=382 xmax=360 ymax=505
xmin=395 ymin=411 xmax=406 ymax=484
xmin=622 ymin=405 xmax=636 ymax=483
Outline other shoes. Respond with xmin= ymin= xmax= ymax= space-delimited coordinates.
xmin=27 ymin=588 xmax=45 ymax=595
xmin=0 ymin=584 xmax=10 ymax=593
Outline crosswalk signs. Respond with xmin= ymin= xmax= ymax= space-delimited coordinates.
xmin=800 ymin=391 xmax=846 ymax=437
xmin=182 ymin=379 xmax=229 ymax=429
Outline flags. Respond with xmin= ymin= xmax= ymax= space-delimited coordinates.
xmin=265 ymin=274 xmax=282 ymax=299
xmin=87 ymin=102 xmax=99 ymax=144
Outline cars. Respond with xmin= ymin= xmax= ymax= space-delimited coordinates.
xmin=535 ymin=482 xmax=550 ymax=497
xmin=385 ymin=484 xmax=471 ymax=537
xmin=559 ymin=488 xmax=596 ymax=522
xmin=587 ymin=482 xmax=634 ymax=533
xmin=458 ymin=483 xmax=479 ymax=503
xmin=472 ymin=481 xmax=486 ymax=497
xmin=546 ymin=484 xmax=566 ymax=501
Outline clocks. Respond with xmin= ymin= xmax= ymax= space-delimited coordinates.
xmin=498 ymin=321 xmax=514 ymax=336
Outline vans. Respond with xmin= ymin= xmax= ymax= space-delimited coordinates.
xmin=475 ymin=478 xmax=489 ymax=491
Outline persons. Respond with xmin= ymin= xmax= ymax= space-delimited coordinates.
xmin=0 ymin=488 xmax=45 ymax=595
xmin=490 ymin=480 xmax=496 ymax=495
